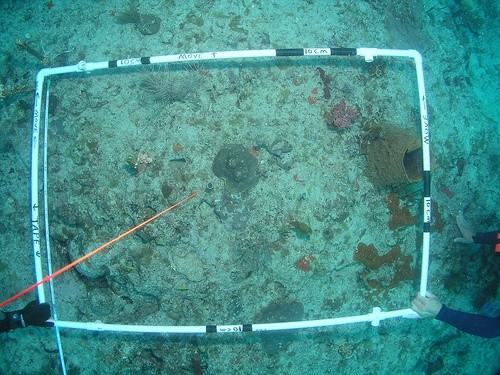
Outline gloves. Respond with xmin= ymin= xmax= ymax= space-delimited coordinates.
xmin=20 ymin=299 xmax=51 ymax=327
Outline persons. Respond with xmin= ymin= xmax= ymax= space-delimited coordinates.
xmin=410 ymin=226 xmax=500 ymax=338
xmin=0 ymin=300 xmax=56 ymax=334
